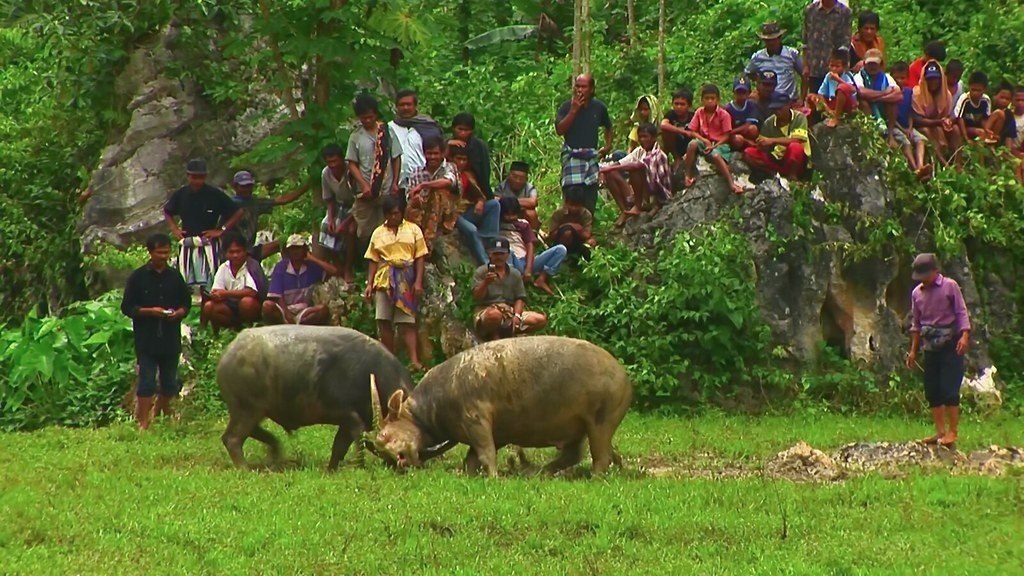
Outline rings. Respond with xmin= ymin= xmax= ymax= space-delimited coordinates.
xmin=516 ymin=325 xmax=520 ymax=328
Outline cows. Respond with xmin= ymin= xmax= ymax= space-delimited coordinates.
xmin=213 ymin=322 xmax=460 ymax=473
xmin=366 ymin=333 xmax=635 ymax=482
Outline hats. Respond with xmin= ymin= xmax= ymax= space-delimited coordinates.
xmin=864 ymin=48 xmax=883 ymax=64
xmin=487 ymin=238 xmax=509 ymax=253
xmin=756 ymin=22 xmax=788 ymax=39
xmin=924 ymin=64 xmax=941 ymax=80
xmin=511 ymin=161 xmax=529 ymax=173
xmin=234 ymin=171 xmax=255 ymax=186
xmin=733 ymin=78 xmax=751 ymax=92
xmin=759 ymin=70 xmax=777 ymax=83
xmin=186 ymin=159 xmax=209 ymax=175
xmin=286 ymin=234 xmax=305 ymax=248
xmin=911 ymin=253 xmax=937 ymax=280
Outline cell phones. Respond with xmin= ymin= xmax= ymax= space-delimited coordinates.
xmin=488 ymin=264 xmax=495 ymax=272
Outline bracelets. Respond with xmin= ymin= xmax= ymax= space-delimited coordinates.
xmin=514 ymin=313 xmax=522 ymax=317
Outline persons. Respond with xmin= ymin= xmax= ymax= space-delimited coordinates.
xmin=554 ymin=75 xmax=613 ymax=247
xmin=601 ymin=0 xmax=1024 ymax=227
xmin=163 ymin=91 xmax=567 ymax=372
xmin=906 ymin=254 xmax=971 ymax=444
xmin=121 ymin=234 xmax=192 ymax=428
xmin=549 ymin=187 xmax=597 ymax=264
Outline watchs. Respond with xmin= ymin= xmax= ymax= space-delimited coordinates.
xmin=221 ymin=224 xmax=231 ymax=232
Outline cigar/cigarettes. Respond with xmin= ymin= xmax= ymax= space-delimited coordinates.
xmin=576 ymin=92 xmax=580 ymax=96
